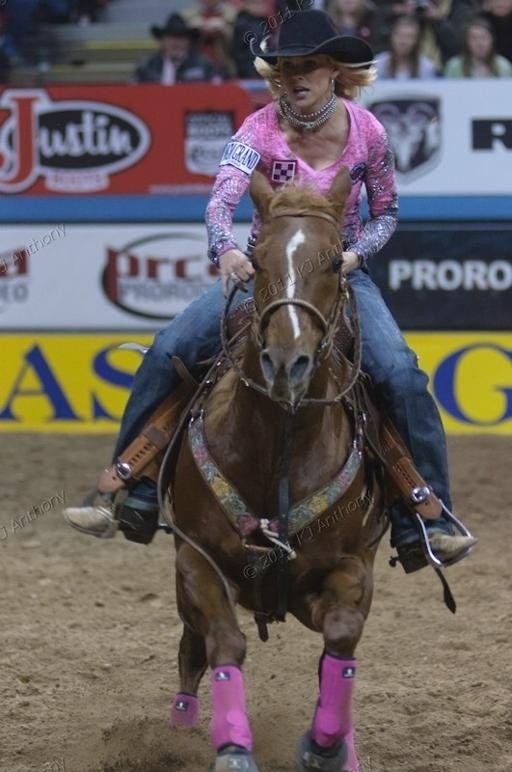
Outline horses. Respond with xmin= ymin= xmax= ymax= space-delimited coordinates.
xmin=163 ymin=163 xmax=395 ymax=772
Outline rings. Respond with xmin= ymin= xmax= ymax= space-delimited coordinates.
xmin=230 ymin=271 xmax=237 ymax=277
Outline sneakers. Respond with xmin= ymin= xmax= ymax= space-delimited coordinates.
xmin=61 ymin=501 xmax=158 ymax=544
xmin=395 ymin=530 xmax=476 ymax=573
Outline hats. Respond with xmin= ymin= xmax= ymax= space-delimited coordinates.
xmin=249 ymin=10 xmax=371 ymax=72
xmin=148 ymin=15 xmax=204 ymax=46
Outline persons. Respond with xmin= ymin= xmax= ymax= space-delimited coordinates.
xmin=127 ymin=0 xmax=512 ymax=84
xmin=63 ymin=8 xmax=480 ymax=575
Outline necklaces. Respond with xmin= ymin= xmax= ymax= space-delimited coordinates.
xmin=278 ymin=92 xmax=337 ymax=131
